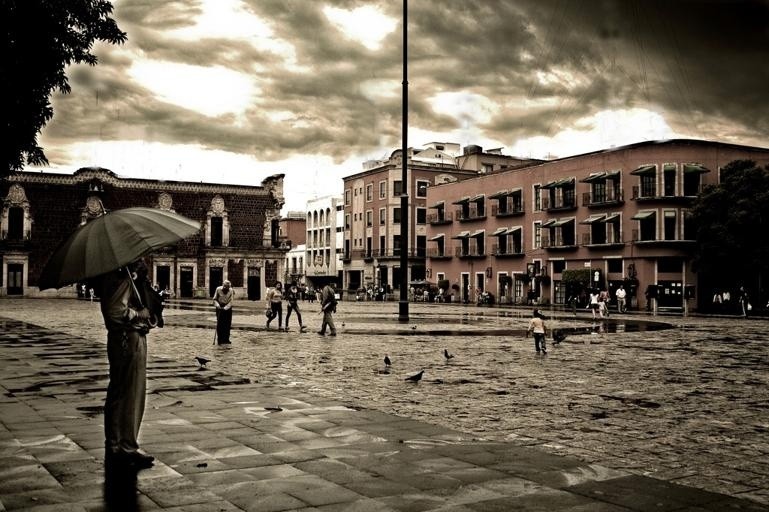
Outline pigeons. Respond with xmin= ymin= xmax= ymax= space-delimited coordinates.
xmin=552 ymin=330 xmax=568 ymax=345
xmin=405 ymin=371 xmax=425 ymax=384
xmin=384 ymin=354 xmax=392 ymax=368
xmin=444 ymin=349 xmax=454 ymax=361
xmin=194 ymin=357 xmax=211 ymax=367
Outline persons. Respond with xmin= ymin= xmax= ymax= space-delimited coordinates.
xmin=525 ymin=308 xmax=548 ymax=354
xmin=284 ymin=283 xmax=489 ymax=306
xmin=317 ymin=279 xmax=337 ymax=336
xmin=98 ymin=257 xmax=156 ymax=466
xmin=266 ymin=281 xmax=285 ymax=332
xmin=285 ymin=281 xmax=307 ymax=330
xmin=567 ymin=283 xmax=628 ymax=321
xmin=212 ymin=279 xmax=234 ymax=345
xmin=712 ymin=283 xmax=750 ymax=317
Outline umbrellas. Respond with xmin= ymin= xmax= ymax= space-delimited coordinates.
xmin=36 ymin=200 xmax=204 ymax=328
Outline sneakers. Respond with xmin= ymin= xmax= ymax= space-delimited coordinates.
xmin=266 ymin=321 xmax=307 ymax=331
xmin=317 ymin=331 xmax=337 ymax=336
xmin=106 ymin=452 xmax=155 ymax=464
xmin=603 ymin=311 xmax=609 ymax=316
xmin=536 ymin=348 xmax=547 ymax=353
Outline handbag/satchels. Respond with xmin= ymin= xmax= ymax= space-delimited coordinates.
xmin=267 ymin=311 xmax=273 ymax=318
xmin=747 ymin=304 xmax=752 ymax=310
xmin=606 ymin=297 xmax=611 ymax=303
xmin=585 ymin=303 xmax=592 ymax=309
